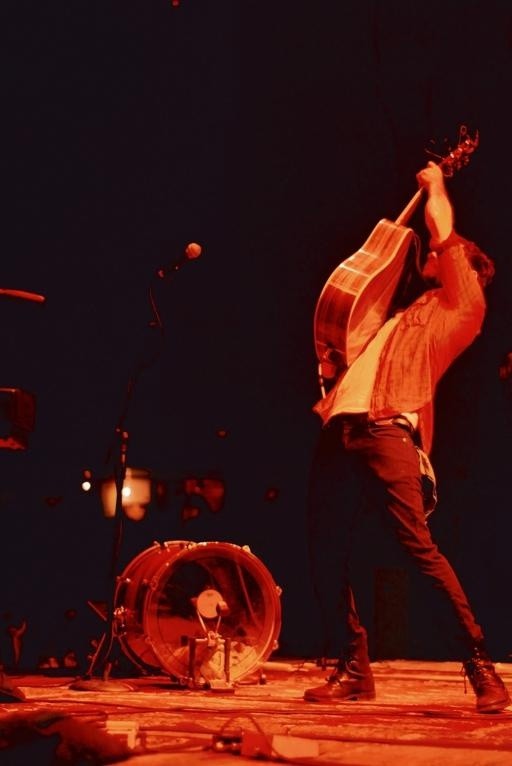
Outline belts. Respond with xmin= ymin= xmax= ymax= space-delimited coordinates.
xmin=374 ymin=417 xmax=411 ymax=431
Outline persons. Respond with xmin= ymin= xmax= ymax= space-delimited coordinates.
xmin=302 ymin=158 xmax=509 ymax=716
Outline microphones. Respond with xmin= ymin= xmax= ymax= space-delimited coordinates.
xmin=154 ymin=242 xmax=202 ymax=281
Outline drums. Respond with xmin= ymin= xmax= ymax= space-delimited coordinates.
xmin=115 ymin=541 xmax=282 ymax=691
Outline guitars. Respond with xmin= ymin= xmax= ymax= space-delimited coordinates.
xmin=314 ymin=124 xmax=481 ymax=368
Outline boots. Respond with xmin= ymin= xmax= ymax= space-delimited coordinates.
xmin=458 ymin=641 xmax=512 ymax=715
xmin=301 ymin=639 xmax=378 ymax=704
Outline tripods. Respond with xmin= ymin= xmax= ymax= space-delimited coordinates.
xmin=80 ymin=281 xmax=168 ymax=686
